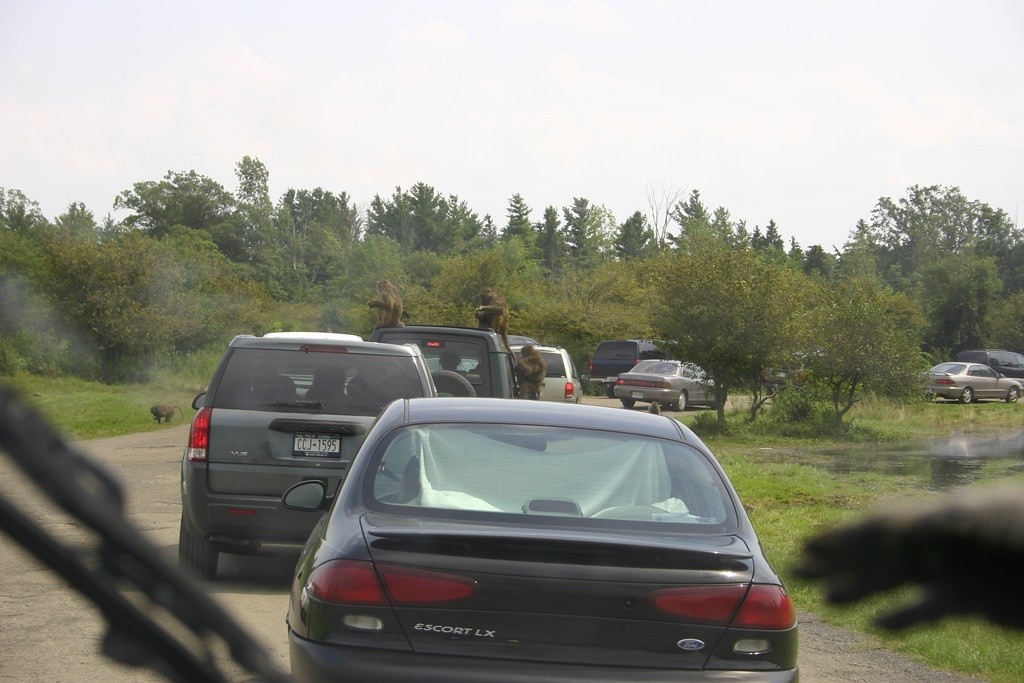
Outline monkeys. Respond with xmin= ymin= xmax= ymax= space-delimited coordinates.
xmin=648 ymin=402 xmax=663 ymax=415
xmin=518 ymin=346 xmax=546 ymax=399
xmin=475 ymin=286 xmax=516 ymax=363
xmin=151 ymin=405 xmax=184 ymax=424
xmin=784 ymin=476 xmax=1024 ymax=631
xmin=367 ymin=278 xmax=412 ymax=329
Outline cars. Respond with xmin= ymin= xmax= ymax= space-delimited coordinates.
xmin=920 ymin=361 xmax=1023 ymax=404
xmin=278 ymin=393 xmax=803 ymax=683
xmin=420 ymin=334 xmax=544 ymax=374
xmin=613 ymin=358 xmax=728 ymax=412
xmin=761 ymin=350 xmax=829 ymax=396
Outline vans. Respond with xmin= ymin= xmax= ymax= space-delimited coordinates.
xmin=508 ymin=343 xmax=584 ymax=404
xmin=358 ymin=324 xmax=515 ymax=400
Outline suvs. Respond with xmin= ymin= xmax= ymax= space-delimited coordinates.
xmin=178 ymin=329 xmax=439 ymax=584
xmin=586 ymin=338 xmax=685 ymax=399
xmin=955 ymin=348 xmax=1024 ymax=397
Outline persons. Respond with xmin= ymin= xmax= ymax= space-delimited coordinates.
xmin=306 ymin=363 xmax=346 ymax=399
xmin=439 ymin=352 xmax=460 ymax=370
